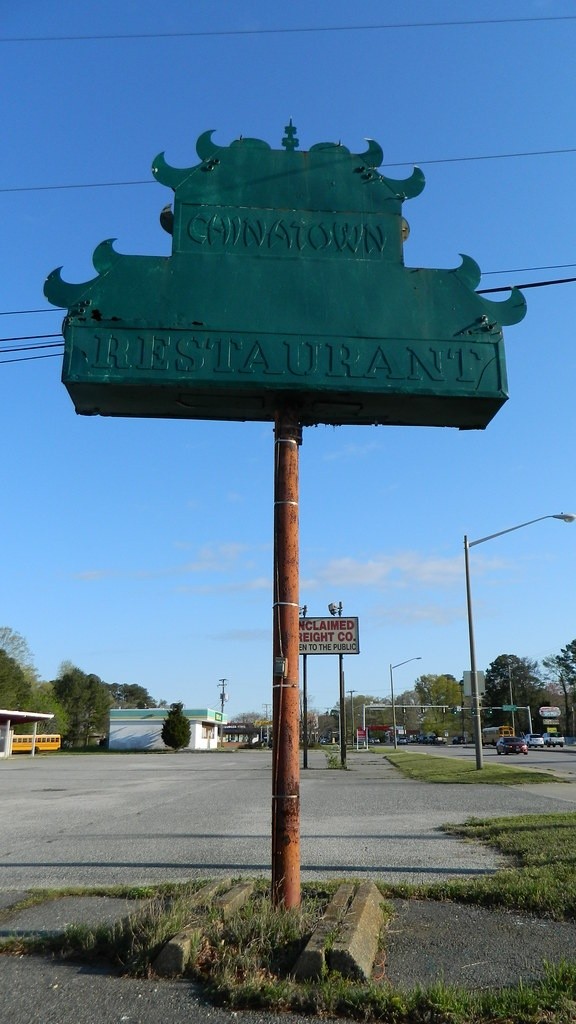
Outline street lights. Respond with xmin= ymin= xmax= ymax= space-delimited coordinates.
xmin=463 ymin=512 xmax=576 ymax=770
xmin=390 ymin=657 xmax=422 ymax=749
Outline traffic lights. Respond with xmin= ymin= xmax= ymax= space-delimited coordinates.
xmin=488 ymin=709 xmax=494 ymax=714
xmin=451 ymin=709 xmax=457 ymax=714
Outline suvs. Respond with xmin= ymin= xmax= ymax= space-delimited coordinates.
xmin=525 ymin=734 xmax=544 ymax=748
xmin=543 ymin=733 xmax=565 ymax=747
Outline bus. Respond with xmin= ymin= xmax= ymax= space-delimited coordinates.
xmin=482 ymin=726 xmax=514 ymax=746
xmin=12 ymin=734 xmax=61 ymax=754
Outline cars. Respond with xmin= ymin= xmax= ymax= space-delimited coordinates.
xmin=496 ymin=737 xmax=528 ymax=755
xmin=369 ymin=736 xmax=467 ymax=746
